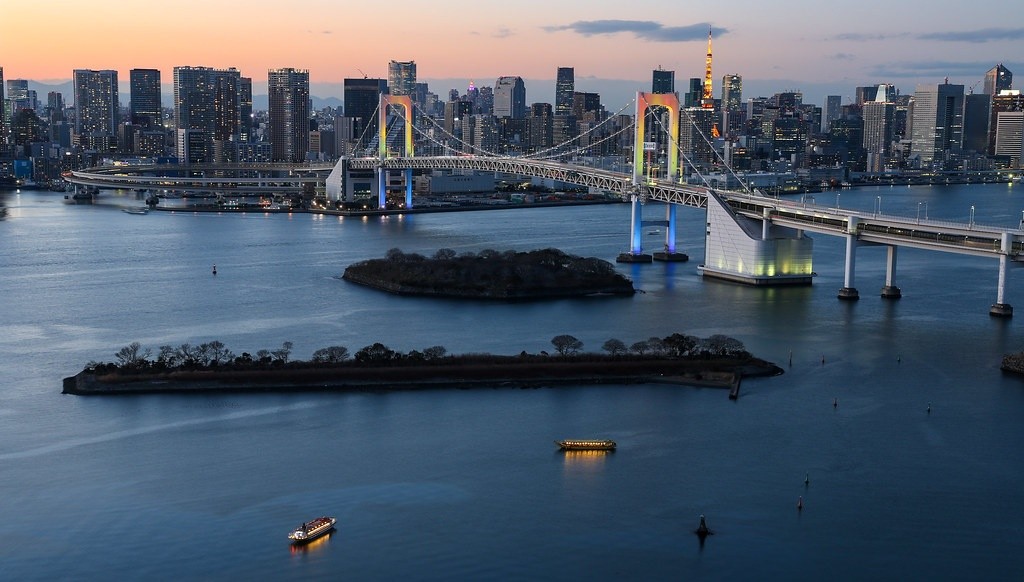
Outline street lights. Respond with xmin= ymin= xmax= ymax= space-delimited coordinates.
xmin=874 ymin=194 xmax=880 ymax=219
xmin=917 ymin=203 xmax=921 ymax=224
xmin=968 ymin=208 xmax=974 ymax=228
xmin=972 ymin=206 xmax=974 ymax=223
xmin=804 ymin=187 xmax=809 ymax=209
xmin=836 ymin=191 xmax=840 ymax=208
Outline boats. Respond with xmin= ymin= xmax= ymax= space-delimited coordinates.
xmin=648 ymin=230 xmax=663 ymax=235
xmin=554 ymin=439 xmax=616 ymax=450
xmin=287 ymin=516 xmax=337 ymax=542
